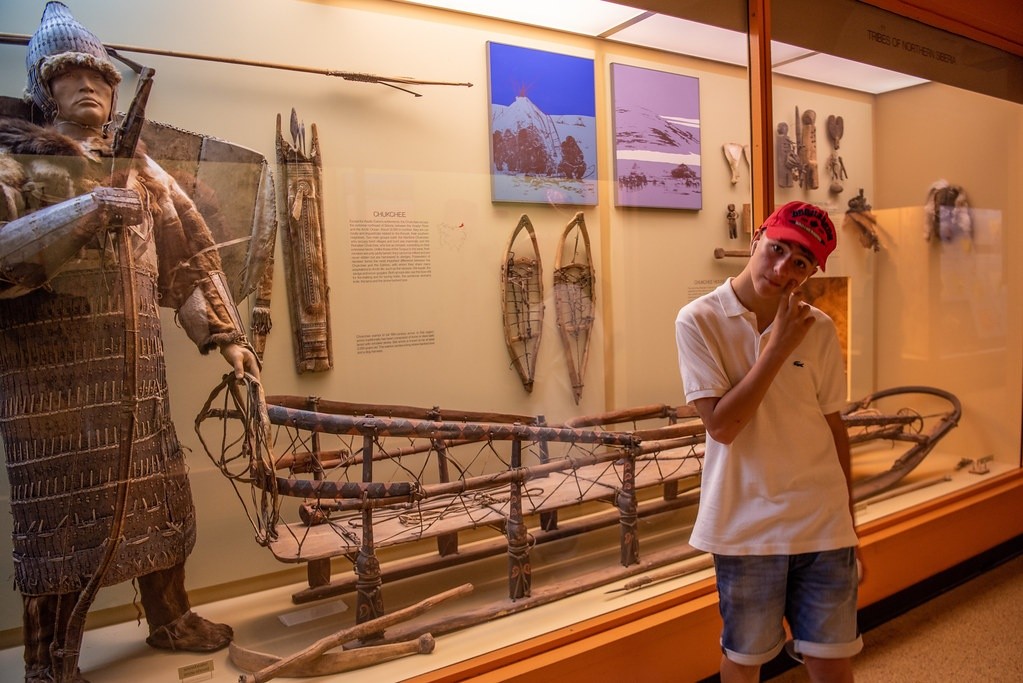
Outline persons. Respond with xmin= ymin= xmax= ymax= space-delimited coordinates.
xmin=675 ymin=202 xmax=864 ymax=683
xmin=0 ymin=0 xmax=262 ymax=682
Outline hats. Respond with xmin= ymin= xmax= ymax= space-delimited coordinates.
xmin=760 ymin=202 xmax=837 ymax=273
